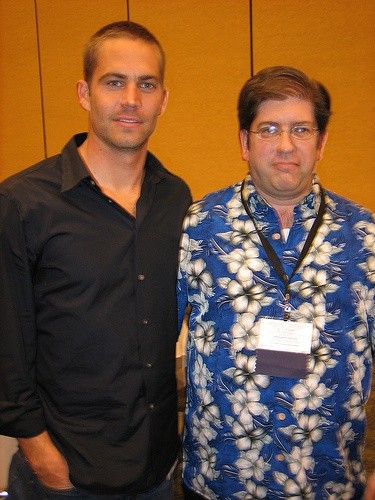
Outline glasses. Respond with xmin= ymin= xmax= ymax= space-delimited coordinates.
xmin=248 ymin=125 xmax=319 ymax=140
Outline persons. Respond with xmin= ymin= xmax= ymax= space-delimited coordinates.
xmin=0 ymin=21 xmax=193 ymax=500
xmin=177 ymin=66 xmax=375 ymax=500
